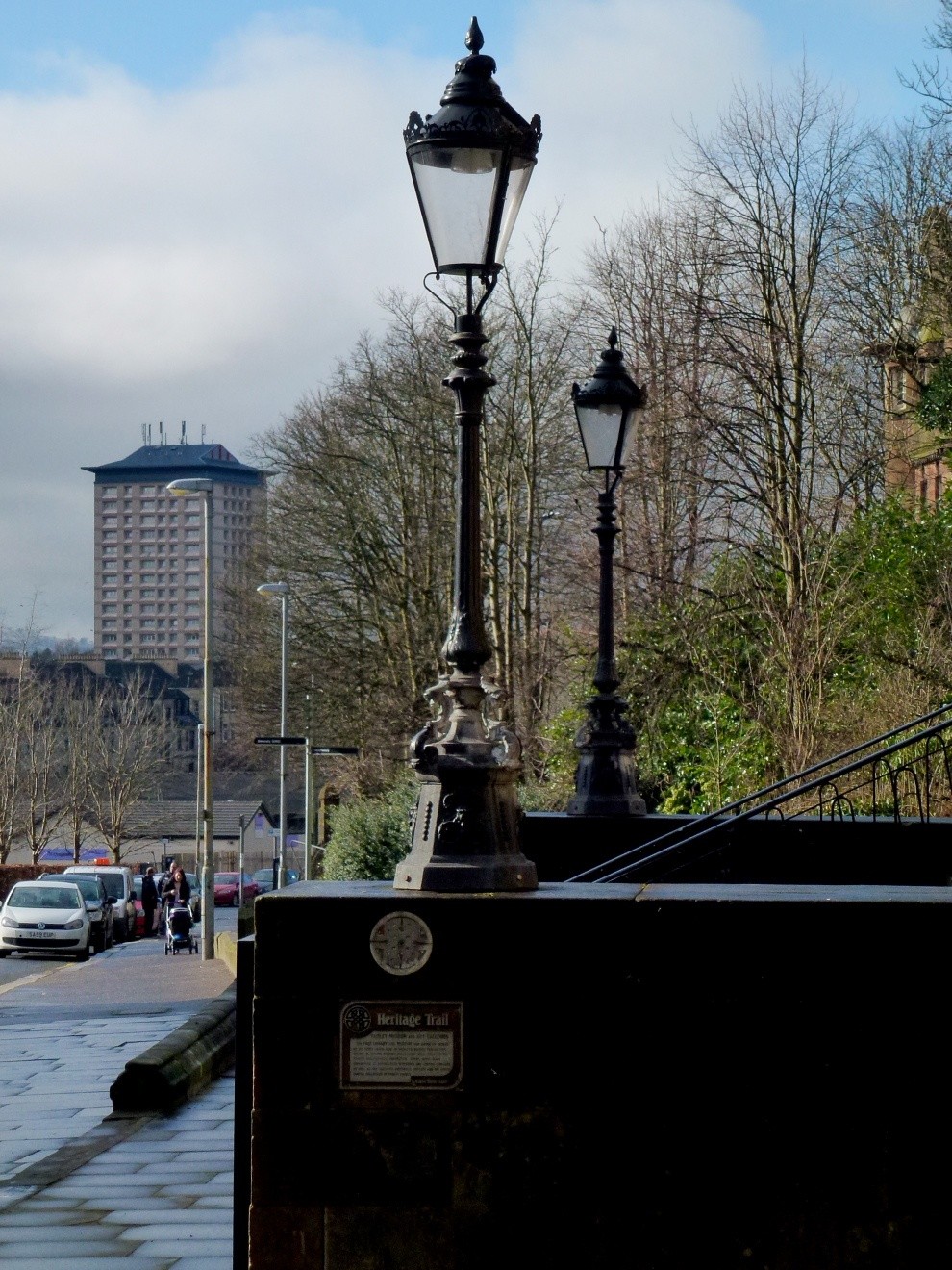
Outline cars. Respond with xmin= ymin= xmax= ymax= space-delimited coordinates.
xmin=0 ymin=880 xmax=99 ymax=963
xmin=214 ymin=871 xmax=259 ymax=908
xmin=252 ymin=867 xmax=299 ymax=896
xmin=130 ymin=870 xmax=202 ymax=938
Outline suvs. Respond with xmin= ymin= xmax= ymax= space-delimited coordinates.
xmin=16 ymin=872 xmax=118 ymax=955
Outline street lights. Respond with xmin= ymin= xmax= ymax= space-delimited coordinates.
xmin=166 ymin=475 xmax=220 ymax=961
xmin=564 ymin=325 xmax=647 ymax=819
xmin=399 ymin=15 xmax=547 ymax=893
xmin=256 ymin=581 xmax=291 ymax=889
xmin=289 ymin=660 xmax=313 ymax=882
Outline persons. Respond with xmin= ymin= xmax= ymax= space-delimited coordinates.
xmin=161 ymin=867 xmax=191 ymax=953
xmin=141 ymin=867 xmax=157 ymax=937
xmin=157 ymin=862 xmax=180 ymax=933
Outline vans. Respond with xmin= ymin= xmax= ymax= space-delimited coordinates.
xmin=63 ymin=857 xmax=135 ymax=944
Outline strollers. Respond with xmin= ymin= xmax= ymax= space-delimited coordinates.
xmin=164 ymin=900 xmax=199 ymax=956
